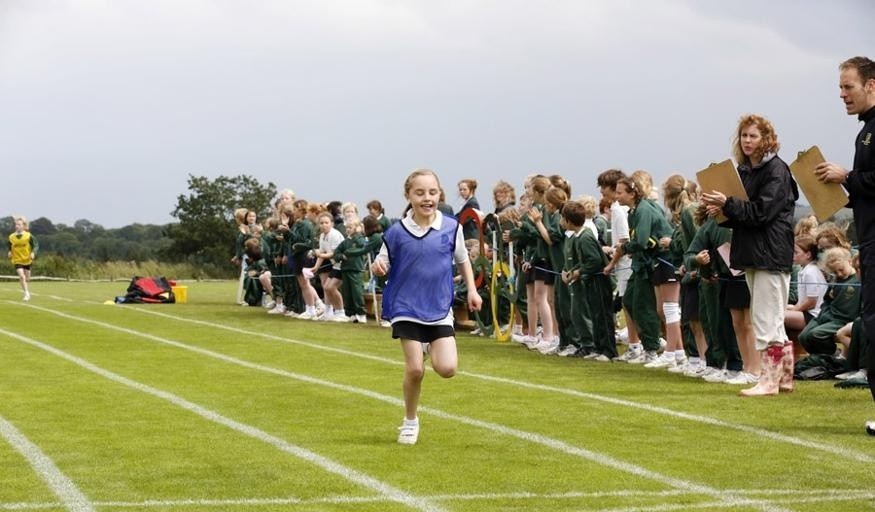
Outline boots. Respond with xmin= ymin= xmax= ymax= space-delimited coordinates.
xmin=739 ymin=340 xmax=794 ymax=396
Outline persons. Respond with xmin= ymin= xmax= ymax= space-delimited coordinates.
xmin=700 ymin=116 xmax=799 ymax=395
xmin=439 ymin=170 xmax=873 ymax=394
xmin=813 ymin=56 xmax=875 ymax=435
xmin=370 ymin=168 xmax=483 ymax=447
xmin=6 ymin=217 xmax=40 ymax=302
xmin=233 ymin=187 xmax=392 ymax=329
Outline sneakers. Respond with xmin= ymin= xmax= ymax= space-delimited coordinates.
xmin=470 ymin=328 xmax=610 ymax=361
xmin=266 ymin=300 xmax=367 ymax=323
xmin=397 ymin=421 xmax=419 ymax=444
xmin=612 ymin=349 xmax=760 ymax=385
